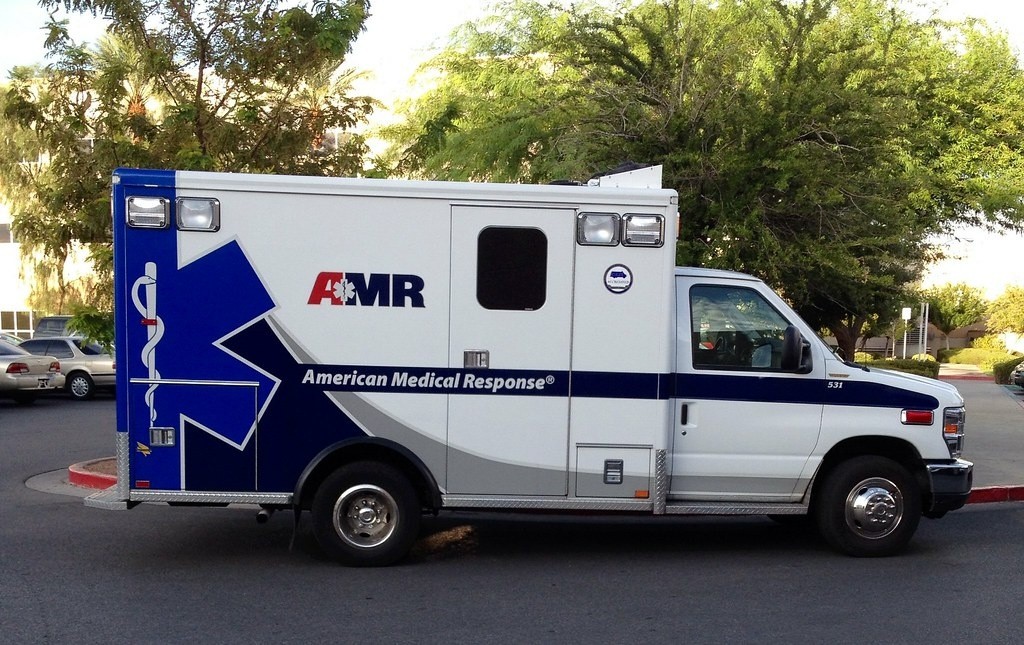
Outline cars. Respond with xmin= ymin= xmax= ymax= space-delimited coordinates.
xmin=1014 ymin=360 xmax=1024 ymax=390
xmin=1 ymin=333 xmax=23 ymax=347
xmin=17 ymin=337 xmax=116 ymax=402
xmin=0 ymin=339 xmax=67 ymax=406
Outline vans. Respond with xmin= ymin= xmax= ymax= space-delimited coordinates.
xmin=33 ymin=315 xmax=81 ymax=340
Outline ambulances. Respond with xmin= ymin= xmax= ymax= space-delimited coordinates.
xmin=82 ymin=166 xmax=975 ymax=559
